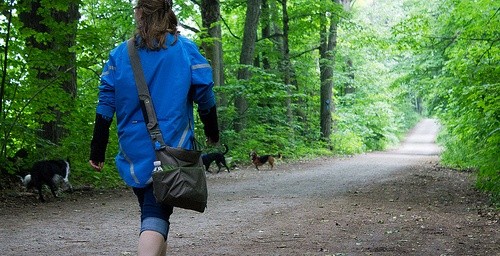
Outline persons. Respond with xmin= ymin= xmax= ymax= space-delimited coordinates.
xmin=87 ymin=0 xmax=223 ymax=256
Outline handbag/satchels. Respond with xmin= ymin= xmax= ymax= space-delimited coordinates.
xmin=153 ymin=143 xmax=208 ymax=213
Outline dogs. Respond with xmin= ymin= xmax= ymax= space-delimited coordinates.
xmin=249 ymin=150 xmax=282 ymax=171
xmin=15 ymin=157 xmax=74 ymax=203
xmin=200 ymin=144 xmax=230 ymax=174
